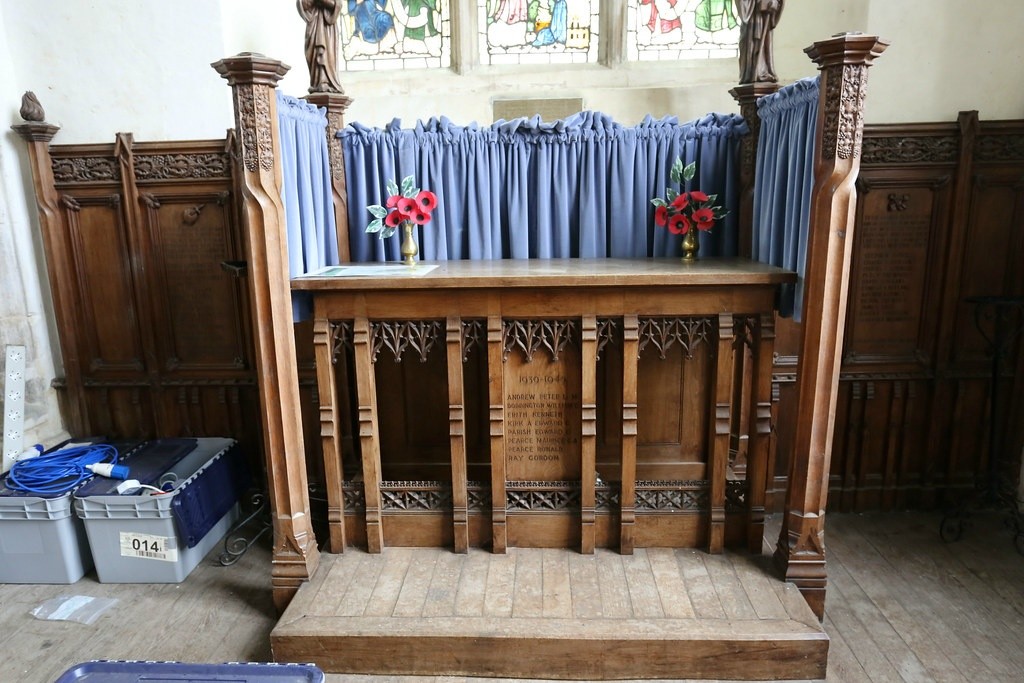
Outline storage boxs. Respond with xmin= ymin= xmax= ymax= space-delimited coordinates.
xmin=0 ymin=437 xmax=241 ymax=585
xmin=54 ymin=659 xmax=326 ymax=683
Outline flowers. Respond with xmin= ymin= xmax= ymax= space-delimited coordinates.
xmin=650 ymin=155 xmax=732 ymax=235
xmin=365 ymin=175 xmax=437 ymax=239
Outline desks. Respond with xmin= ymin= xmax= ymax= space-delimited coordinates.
xmin=290 ymin=258 xmax=798 ymax=556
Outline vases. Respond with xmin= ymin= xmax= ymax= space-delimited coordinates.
xmin=402 ymin=224 xmax=417 ymax=265
xmin=679 ymin=225 xmax=699 ymax=263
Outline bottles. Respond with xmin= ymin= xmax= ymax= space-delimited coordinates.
xmin=14 ymin=444 xmax=44 ymax=461
xmin=85 ymin=462 xmax=130 ymax=480
